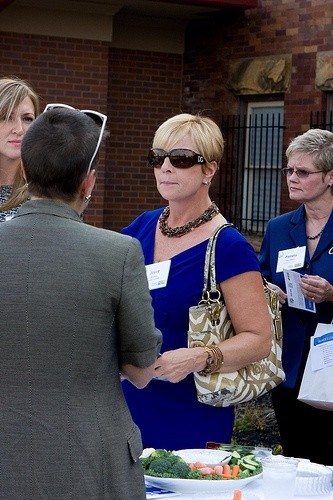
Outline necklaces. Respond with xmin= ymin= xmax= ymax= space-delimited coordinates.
xmin=305 ymin=226 xmax=325 ymax=239
xmin=158 ymin=200 xmax=218 ymax=238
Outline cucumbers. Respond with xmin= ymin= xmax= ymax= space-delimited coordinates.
xmin=227 ymin=450 xmax=259 ymax=472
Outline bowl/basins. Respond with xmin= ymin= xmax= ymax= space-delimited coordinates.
xmin=174 ymin=448 xmax=232 ymax=468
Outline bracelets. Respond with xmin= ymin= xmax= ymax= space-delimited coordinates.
xmin=196 ymin=345 xmax=224 ymax=377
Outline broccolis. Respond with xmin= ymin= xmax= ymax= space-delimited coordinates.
xmin=138 ymin=448 xmax=191 ymax=475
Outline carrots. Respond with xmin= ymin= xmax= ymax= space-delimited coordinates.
xmin=189 ymin=461 xmax=240 ymax=479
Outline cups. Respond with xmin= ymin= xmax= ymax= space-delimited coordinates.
xmin=259 ymin=457 xmax=300 ymax=500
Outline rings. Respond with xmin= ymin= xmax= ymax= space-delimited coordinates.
xmin=311 ymin=297 xmax=315 ymax=301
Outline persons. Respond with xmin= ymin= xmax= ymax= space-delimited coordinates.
xmin=117 ymin=112 xmax=272 ymax=452
xmin=258 ymin=128 xmax=333 ymax=466
xmin=0 ymin=102 xmax=163 ymax=500
xmin=0 ymin=73 xmax=41 ymax=224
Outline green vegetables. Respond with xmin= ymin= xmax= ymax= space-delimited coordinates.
xmin=145 ymin=468 xmax=223 ymax=480
xmin=237 ymin=461 xmax=262 ymax=479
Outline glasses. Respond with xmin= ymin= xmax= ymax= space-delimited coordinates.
xmin=147 ymin=148 xmax=206 ymax=170
xmin=42 ymin=103 xmax=108 ymax=175
xmin=281 ymin=167 xmax=331 ymax=179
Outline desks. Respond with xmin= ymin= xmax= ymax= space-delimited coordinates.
xmin=145 ymin=449 xmax=333 ymax=500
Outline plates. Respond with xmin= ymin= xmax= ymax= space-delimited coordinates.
xmin=297 ymin=462 xmax=333 ymax=497
xmin=144 ymin=470 xmax=264 ymax=494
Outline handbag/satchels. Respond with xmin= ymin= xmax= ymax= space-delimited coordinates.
xmin=186 ymin=288 xmax=288 ymax=409
